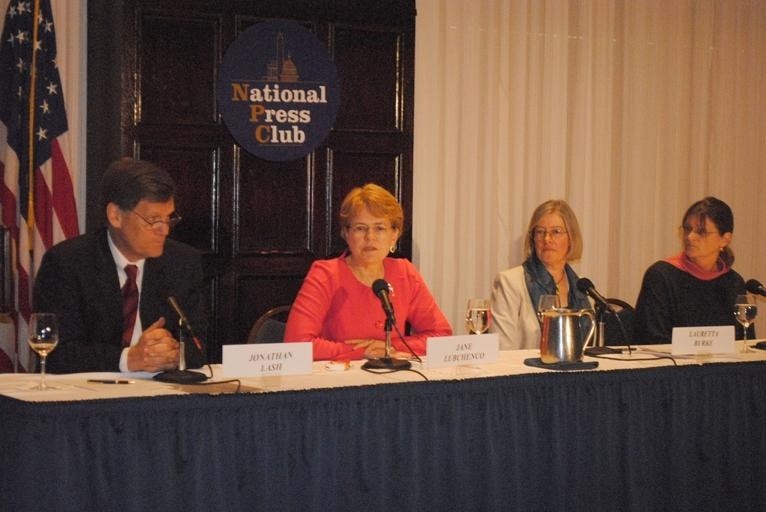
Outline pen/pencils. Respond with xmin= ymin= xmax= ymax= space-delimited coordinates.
xmin=88 ymin=379 xmax=136 ymax=385
xmin=618 ymin=347 xmax=636 ymax=351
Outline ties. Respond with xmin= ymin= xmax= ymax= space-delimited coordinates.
xmin=117 ymin=264 xmax=139 ymax=347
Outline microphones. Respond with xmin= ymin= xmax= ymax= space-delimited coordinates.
xmin=372 ymin=279 xmax=396 ymax=325
xmin=746 ymin=279 xmax=766 ymax=297
xmin=576 ymin=278 xmax=614 ymax=312
xmin=156 ymin=281 xmax=195 ymax=338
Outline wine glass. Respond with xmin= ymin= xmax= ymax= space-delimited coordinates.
xmin=732 ymin=293 xmax=758 ymax=356
xmin=464 ymin=298 xmax=492 ymax=335
xmin=536 ymin=293 xmax=561 ymax=324
xmin=26 ymin=311 xmax=59 ymax=392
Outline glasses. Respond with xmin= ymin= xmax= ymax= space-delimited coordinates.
xmin=679 ymin=226 xmax=718 ymax=237
xmin=533 ymin=230 xmax=566 ymax=240
xmin=348 ymin=223 xmax=393 ymax=235
xmin=130 ymin=208 xmax=182 ymax=231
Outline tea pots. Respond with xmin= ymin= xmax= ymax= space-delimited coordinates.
xmin=537 ymin=306 xmax=598 ymax=365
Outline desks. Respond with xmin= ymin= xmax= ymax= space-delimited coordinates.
xmin=0 ymin=336 xmax=764 ymax=511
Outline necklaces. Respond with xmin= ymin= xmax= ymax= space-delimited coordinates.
xmin=545 ymin=266 xmax=570 ymax=294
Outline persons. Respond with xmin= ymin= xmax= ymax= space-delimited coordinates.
xmin=282 ymin=182 xmax=453 ymax=363
xmin=33 ymin=156 xmax=210 ymax=374
xmin=487 ymin=198 xmax=599 ymax=351
xmin=636 ymin=195 xmax=756 ymax=344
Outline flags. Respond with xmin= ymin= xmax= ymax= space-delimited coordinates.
xmin=1 ymin=0 xmax=80 ymax=375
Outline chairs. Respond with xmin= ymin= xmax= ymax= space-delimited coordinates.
xmin=242 ymin=303 xmax=292 ymax=345
xmin=598 ymin=292 xmax=639 ymax=345
